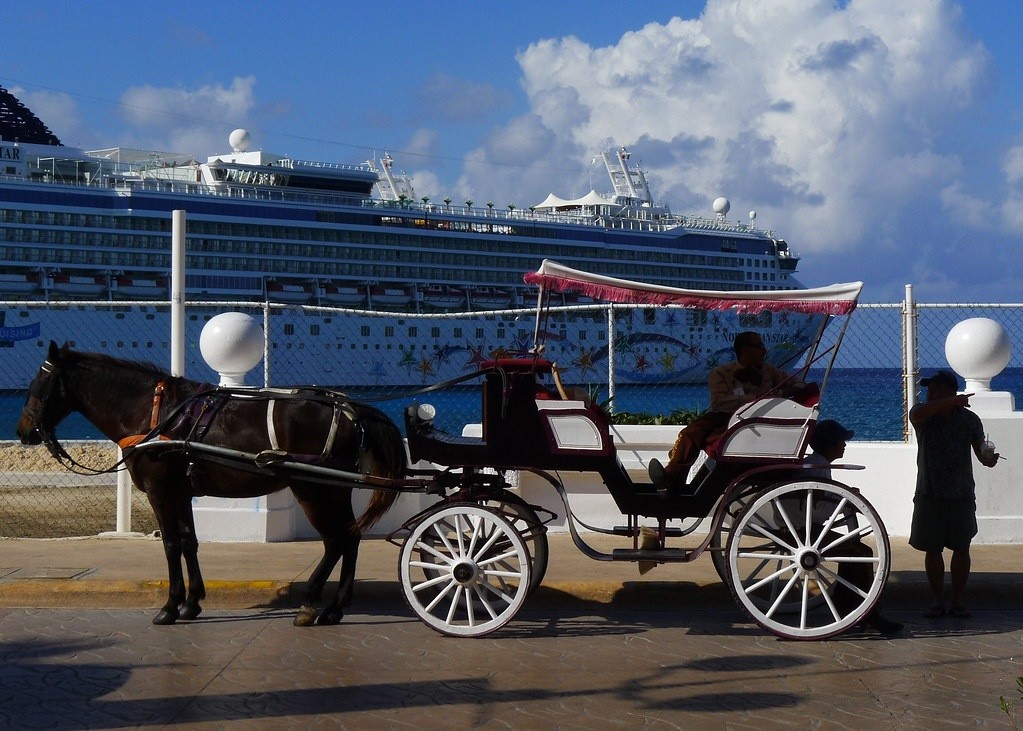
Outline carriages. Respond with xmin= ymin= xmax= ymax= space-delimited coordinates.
xmin=17 ymin=258 xmax=894 ymax=643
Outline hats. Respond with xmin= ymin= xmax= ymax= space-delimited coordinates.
xmin=920 ymin=371 xmax=958 ymax=391
xmin=810 ymin=420 xmax=854 ymax=450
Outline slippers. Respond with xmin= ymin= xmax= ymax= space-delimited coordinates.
xmin=949 ymin=606 xmax=970 ymax=618
xmin=924 ymin=606 xmax=946 ymax=618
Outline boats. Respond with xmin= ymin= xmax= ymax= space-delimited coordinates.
xmin=471 ymin=292 xmax=512 ymax=310
xmin=0 ymin=270 xmax=39 ymax=296
xmin=322 ymin=287 xmax=366 ymax=307
xmin=111 ymin=277 xmax=168 ymax=301
xmin=524 ymin=296 xmax=563 ymax=317
xmin=267 ymin=284 xmax=314 ymax=305
xmin=50 ymin=273 xmax=106 ymax=299
xmin=370 ymin=288 xmax=413 ymax=308
xmin=419 ymin=292 xmax=466 ymax=312
xmin=565 ymin=297 xmax=599 ymax=317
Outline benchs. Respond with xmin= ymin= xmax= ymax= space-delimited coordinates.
xmin=702 ymin=380 xmax=820 ymax=468
xmin=483 ymin=350 xmax=616 ymax=459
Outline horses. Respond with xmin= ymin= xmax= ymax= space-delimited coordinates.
xmin=18 ymin=340 xmax=408 ymax=627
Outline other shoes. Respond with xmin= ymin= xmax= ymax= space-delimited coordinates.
xmin=871 ymin=616 xmax=903 ymax=634
xmin=649 ymin=458 xmax=690 ymax=487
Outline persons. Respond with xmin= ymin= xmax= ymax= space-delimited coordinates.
xmin=773 ymin=420 xmax=880 ymax=619
xmin=648 ymin=332 xmax=806 ymax=493
xmin=909 ymin=371 xmax=999 ymax=628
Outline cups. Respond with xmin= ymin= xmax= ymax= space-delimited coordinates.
xmin=980 ymin=441 xmax=995 ymax=460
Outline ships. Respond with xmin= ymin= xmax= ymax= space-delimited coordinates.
xmin=0 ymin=84 xmax=836 ymax=393
